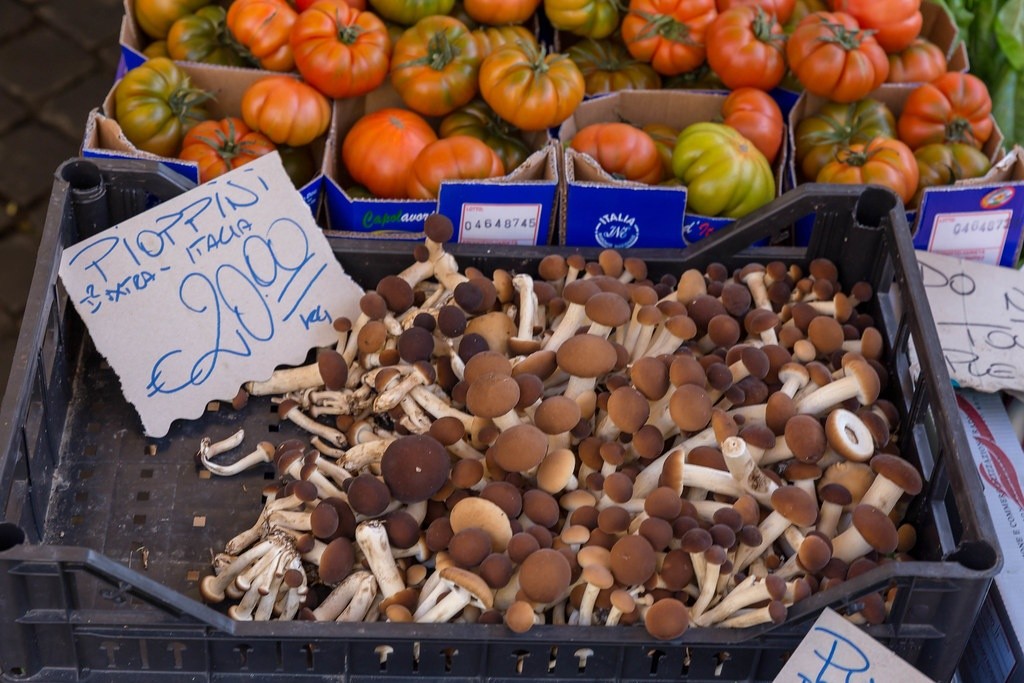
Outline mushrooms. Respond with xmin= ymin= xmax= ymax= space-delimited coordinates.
xmin=196 ymin=214 xmax=922 ymax=673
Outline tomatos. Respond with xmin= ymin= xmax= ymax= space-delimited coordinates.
xmin=116 ymin=0 xmax=1005 ymax=223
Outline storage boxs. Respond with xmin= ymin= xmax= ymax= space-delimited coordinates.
xmin=0 ymin=0 xmax=1024 ymax=683
